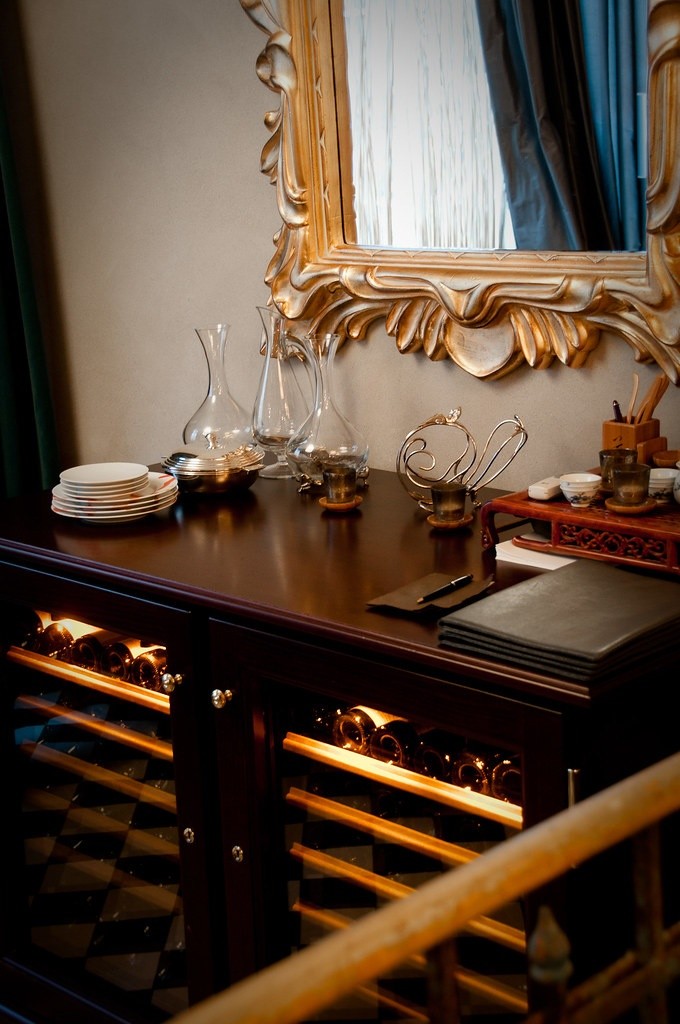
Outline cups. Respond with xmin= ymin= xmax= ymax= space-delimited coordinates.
xmin=326 ymin=462 xmax=356 ymax=503
xmin=599 ymin=449 xmax=637 ymax=487
xmin=430 ymin=483 xmax=464 ymax=519
xmin=616 ymin=468 xmax=648 ymax=502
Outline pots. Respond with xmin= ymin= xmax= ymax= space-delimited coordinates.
xmin=158 ymin=436 xmax=264 ymax=495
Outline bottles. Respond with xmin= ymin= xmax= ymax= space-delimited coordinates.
xmin=490 ymin=756 xmax=528 ymax=800
xmin=102 ymin=637 xmax=160 ymax=677
xmin=408 ymin=727 xmax=458 ymax=777
xmin=17 ymin=610 xmax=61 ymax=649
xmin=43 ymin=618 xmax=97 ymax=660
xmin=134 ymin=646 xmax=169 ymax=687
xmin=335 ymin=707 xmax=402 ymax=755
xmin=451 ymin=738 xmax=516 ymax=791
xmin=74 ymin=628 xmax=121 ymax=670
xmin=365 ymin=716 xmax=425 ymax=765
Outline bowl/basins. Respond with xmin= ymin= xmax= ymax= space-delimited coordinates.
xmin=646 ymin=469 xmax=679 ymax=499
xmin=560 ymin=475 xmax=599 ymax=508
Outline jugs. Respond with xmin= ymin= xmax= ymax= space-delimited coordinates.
xmin=181 ymin=323 xmax=260 ymax=448
xmin=293 ymin=335 xmax=369 ymax=479
xmin=251 ymin=307 xmax=311 ymax=451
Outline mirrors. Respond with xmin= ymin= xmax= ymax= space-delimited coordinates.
xmin=238 ymin=0 xmax=680 ymax=388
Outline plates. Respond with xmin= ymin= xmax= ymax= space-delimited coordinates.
xmin=319 ymin=496 xmax=363 ymax=509
xmin=52 ymin=462 xmax=179 ymax=524
xmin=608 ymin=498 xmax=655 ymax=511
xmin=425 ymin=513 xmax=474 ymax=527
xmin=598 ymin=486 xmax=619 ymax=495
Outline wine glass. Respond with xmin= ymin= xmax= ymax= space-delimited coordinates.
xmin=255 ymin=396 xmax=295 ymax=478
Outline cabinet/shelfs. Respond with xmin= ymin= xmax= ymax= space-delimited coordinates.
xmin=0 ymin=438 xmax=680 ymax=1024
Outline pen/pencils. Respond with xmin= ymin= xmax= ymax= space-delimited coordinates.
xmin=417 ymin=573 xmax=475 ymax=604
